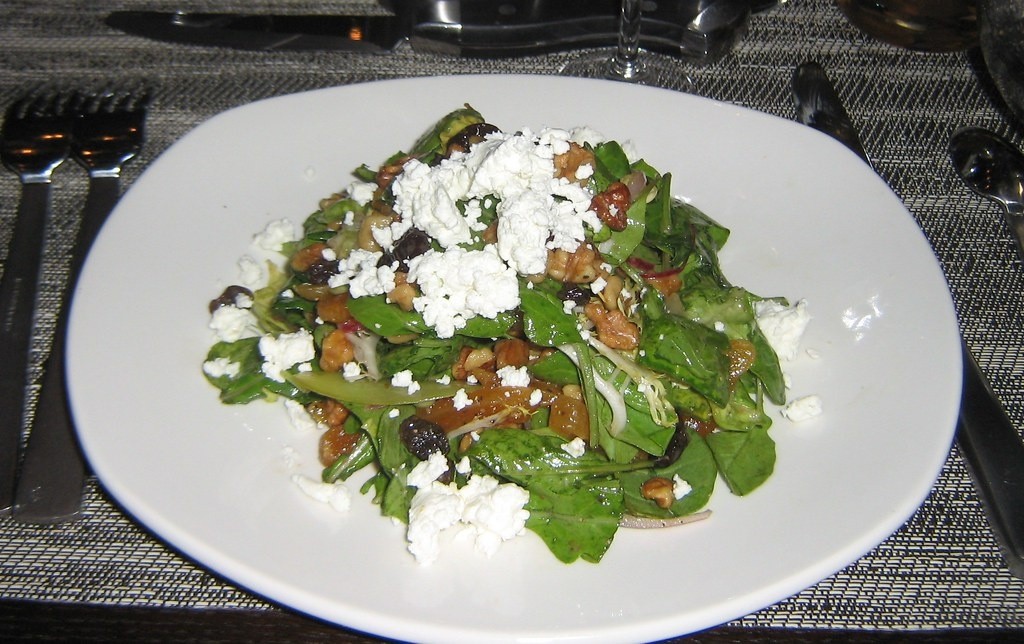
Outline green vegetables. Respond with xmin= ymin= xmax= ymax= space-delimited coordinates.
xmin=204 ymin=109 xmax=789 ymax=562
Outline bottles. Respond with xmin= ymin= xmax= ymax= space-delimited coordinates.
xmin=836 ymin=0 xmax=981 ymax=52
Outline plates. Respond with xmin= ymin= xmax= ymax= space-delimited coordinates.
xmin=66 ymin=72 xmax=961 ymax=643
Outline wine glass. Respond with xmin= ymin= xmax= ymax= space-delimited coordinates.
xmin=562 ymin=1 xmax=695 ymax=97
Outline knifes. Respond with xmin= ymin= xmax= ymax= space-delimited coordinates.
xmin=102 ymin=1 xmax=752 ymax=69
xmin=790 ymin=60 xmax=1024 ymax=581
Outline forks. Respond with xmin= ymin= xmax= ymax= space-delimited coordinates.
xmin=12 ymin=87 xmax=152 ymax=528
xmin=0 ymin=93 xmax=78 ymax=521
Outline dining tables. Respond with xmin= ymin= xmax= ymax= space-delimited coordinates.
xmin=0 ymin=0 xmax=1024 ymax=633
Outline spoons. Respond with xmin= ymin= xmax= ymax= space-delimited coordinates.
xmin=949 ymin=126 xmax=1024 ymax=268
